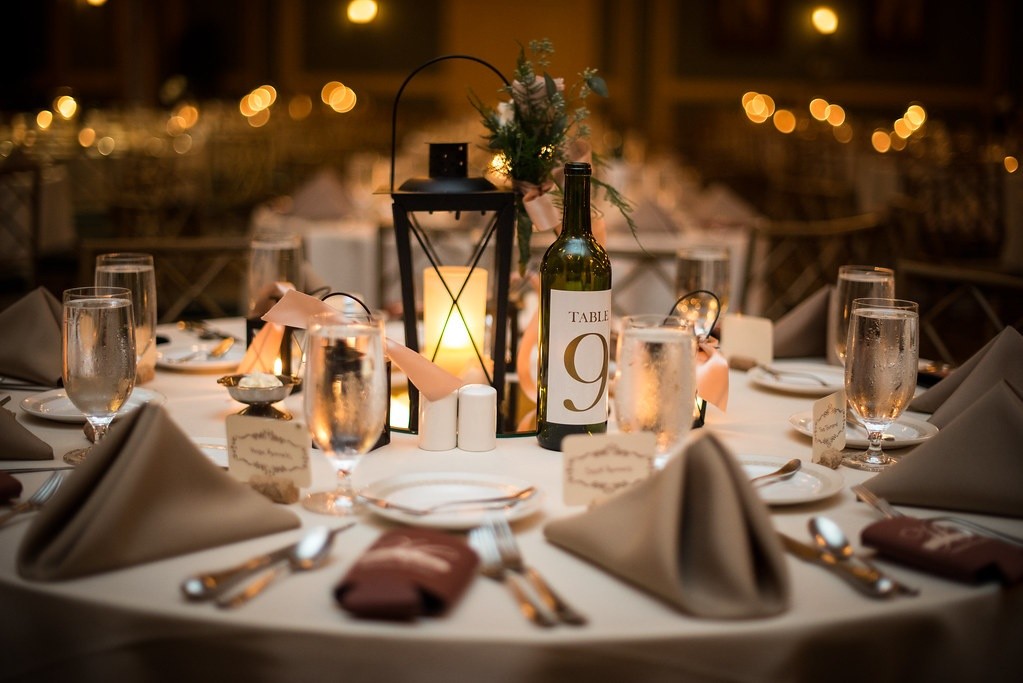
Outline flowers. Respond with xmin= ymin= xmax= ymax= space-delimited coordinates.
xmin=469 ymin=34 xmax=662 ymax=269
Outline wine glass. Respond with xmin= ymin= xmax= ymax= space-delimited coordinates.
xmin=305 ymin=310 xmax=387 ymax=517
xmin=835 ymin=266 xmax=919 ymax=473
xmin=62 ymin=287 xmax=137 ymax=465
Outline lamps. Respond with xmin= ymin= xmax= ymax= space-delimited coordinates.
xmin=388 ymin=55 xmax=529 ymax=439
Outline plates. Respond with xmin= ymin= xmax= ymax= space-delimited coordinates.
xmin=21 ymin=387 xmax=168 ymax=422
xmin=735 ymin=453 xmax=842 ymax=505
xmin=748 ymin=362 xmax=846 ymax=395
xmin=787 ymin=409 xmax=939 ymax=448
xmin=359 ymin=473 xmax=544 ymax=528
xmin=153 ymin=342 xmax=255 ymax=370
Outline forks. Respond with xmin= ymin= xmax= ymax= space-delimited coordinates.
xmin=466 ymin=521 xmax=587 ymax=626
xmin=850 ymin=484 xmax=1022 ymax=549
xmin=0 ymin=471 xmax=63 ymax=526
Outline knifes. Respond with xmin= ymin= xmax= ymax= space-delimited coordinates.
xmin=776 ymin=530 xmax=893 ymax=594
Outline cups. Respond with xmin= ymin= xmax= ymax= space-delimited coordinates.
xmin=245 ymin=243 xmax=303 ymax=351
xmin=96 ymin=252 xmax=158 ymax=387
xmin=613 ymin=315 xmax=695 ymax=457
xmin=674 ymin=239 xmax=734 ymax=336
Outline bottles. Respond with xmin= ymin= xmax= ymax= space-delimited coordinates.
xmin=460 ymin=383 xmax=498 ymax=452
xmin=418 ymin=390 xmax=457 ymax=450
xmin=536 ymin=163 xmax=613 ymax=452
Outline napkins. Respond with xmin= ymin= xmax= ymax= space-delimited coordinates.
xmin=0 ymin=287 xmax=66 ymax=387
xmin=904 ymin=325 xmax=1023 ymax=432
xmin=0 ymin=407 xmax=55 ymax=461
xmin=847 ymin=380 xmax=1023 ymax=508
xmin=17 ymin=397 xmax=305 ymax=575
xmin=764 ymin=284 xmax=832 ymax=358
xmin=542 ymin=426 xmax=794 ymax=620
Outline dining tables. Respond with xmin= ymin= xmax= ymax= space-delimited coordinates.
xmin=248 ymin=210 xmax=766 ymax=334
xmin=0 ymin=315 xmax=1023 ymax=683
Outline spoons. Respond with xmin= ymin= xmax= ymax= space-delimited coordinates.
xmin=165 ymin=336 xmax=235 ymax=364
xmin=808 ymin=516 xmax=921 ymax=598
xmin=212 ymin=526 xmax=331 ymax=609
xmin=748 ymin=459 xmax=800 ymax=486
